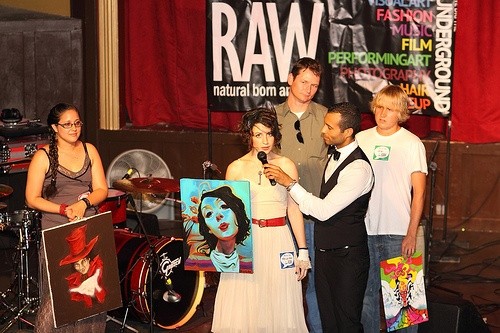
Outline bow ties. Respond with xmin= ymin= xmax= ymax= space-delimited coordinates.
xmin=327 ymin=145 xmax=341 ymax=161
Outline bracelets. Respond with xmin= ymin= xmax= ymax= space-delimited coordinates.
xmin=81 ymin=198 xmax=91 ymax=209
xmin=60 ymin=204 xmax=69 ymax=215
xmin=285 ymin=180 xmax=297 ymax=192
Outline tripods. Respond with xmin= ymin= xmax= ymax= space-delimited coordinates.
xmin=0 ymin=220 xmax=40 ymax=333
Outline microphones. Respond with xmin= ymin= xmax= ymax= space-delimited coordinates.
xmin=122 ymin=167 xmax=135 ymax=181
xmin=257 ymin=151 xmax=276 ymax=186
xmin=205 ymin=160 xmax=222 ymax=177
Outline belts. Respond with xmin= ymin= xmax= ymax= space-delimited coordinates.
xmin=252 ymin=217 xmax=287 ymax=227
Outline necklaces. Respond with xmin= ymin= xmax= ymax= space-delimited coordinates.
xmin=256 ymin=168 xmax=263 ymax=184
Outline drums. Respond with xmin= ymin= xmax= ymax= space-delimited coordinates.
xmin=106 ymin=228 xmax=207 ymax=329
xmin=97 ymin=189 xmax=127 ymax=230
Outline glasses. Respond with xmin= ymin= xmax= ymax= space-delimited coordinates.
xmin=58 ymin=120 xmax=84 ymax=128
xmin=294 ymin=120 xmax=304 ymax=144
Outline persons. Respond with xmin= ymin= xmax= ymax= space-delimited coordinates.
xmin=263 ymin=101 xmax=375 ymax=333
xmin=25 ymin=102 xmax=108 ymax=333
xmin=356 ymin=86 xmax=429 ymax=333
xmin=272 ymin=56 xmax=328 ymax=333
xmin=211 ymin=108 xmax=312 ymax=333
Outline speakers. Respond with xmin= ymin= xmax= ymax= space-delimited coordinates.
xmin=416 ymin=298 xmax=493 ymax=333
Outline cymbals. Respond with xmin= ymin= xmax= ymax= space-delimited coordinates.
xmin=112 ymin=177 xmax=181 ymax=194
